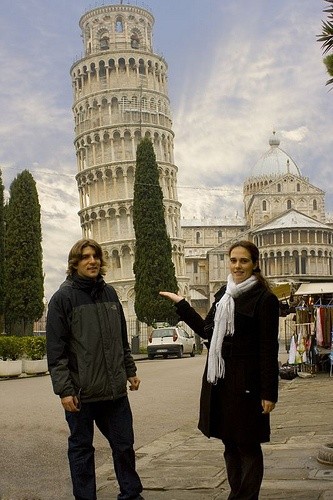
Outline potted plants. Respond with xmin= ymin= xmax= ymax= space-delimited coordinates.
xmin=0 ymin=335 xmax=48 ymax=377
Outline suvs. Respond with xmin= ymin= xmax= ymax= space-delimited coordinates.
xmin=147 ymin=324 xmax=203 ymax=359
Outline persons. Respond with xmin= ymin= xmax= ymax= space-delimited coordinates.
xmin=160 ymin=240 xmax=279 ymax=500
xmin=45 ymin=238 xmax=144 ymax=500
